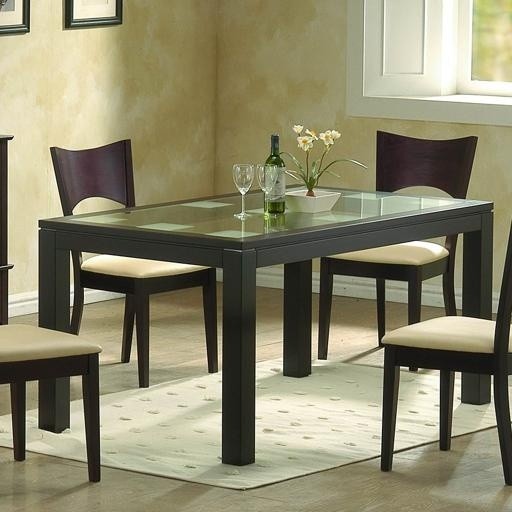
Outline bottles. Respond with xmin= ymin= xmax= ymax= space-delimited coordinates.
xmin=264 ymin=134 xmax=286 ymax=214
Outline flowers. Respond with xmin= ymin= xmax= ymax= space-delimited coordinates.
xmin=278 ymin=125 xmax=369 ymax=196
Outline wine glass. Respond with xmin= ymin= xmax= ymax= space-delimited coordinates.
xmin=232 ymin=163 xmax=277 ymax=218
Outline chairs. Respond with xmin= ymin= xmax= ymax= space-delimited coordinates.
xmin=50 ymin=140 xmax=218 ymax=388
xmin=318 ymin=130 xmax=479 ymax=360
xmin=1 ymin=325 xmax=103 ymax=483
xmin=380 ymin=221 xmax=511 ymax=485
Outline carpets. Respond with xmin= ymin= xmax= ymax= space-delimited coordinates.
xmin=1 ymin=358 xmax=512 ymax=490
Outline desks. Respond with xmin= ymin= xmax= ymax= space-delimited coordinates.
xmin=36 ymin=184 xmax=493 ymax=465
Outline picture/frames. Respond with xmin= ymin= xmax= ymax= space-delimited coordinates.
xmin=0 ymin=0 xmax=31 ymax=34
xmin=65 ymin=0 xmax=122 ymax=28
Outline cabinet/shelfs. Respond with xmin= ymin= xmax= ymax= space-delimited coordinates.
xmin=0 ymin=136 xmax=13 ymax=325
